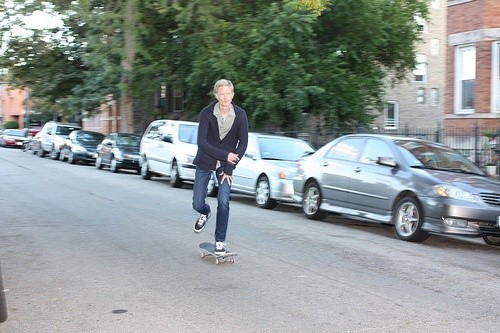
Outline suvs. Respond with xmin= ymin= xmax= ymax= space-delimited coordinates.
xmin=33 ymin=121 xmax=82 ymax=159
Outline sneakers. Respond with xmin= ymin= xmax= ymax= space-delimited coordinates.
xmin=193 ymin=211 xmax=212 ymax=232
xmin=214 ymin=242 xmax=226 ymax=254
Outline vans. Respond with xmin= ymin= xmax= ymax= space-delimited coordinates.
xmin=138 ymin=120 xmax=202 ymax=185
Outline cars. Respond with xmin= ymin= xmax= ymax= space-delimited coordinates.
xmin=291 ymin=133 xmax=500 ymax=242
xmin=28 ymin=126 xmax=41 ymax=135
xmin=60 ymin=130 xmax=106 ymax=164
xmin=205 ymin=132 xmax=317 ymax=209
xmin=0 ymin=129 xmax=32 ymax=149
xmin=95 ymin=132 xmax=143 ymax=173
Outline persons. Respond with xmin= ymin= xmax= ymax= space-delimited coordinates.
xmin=193 ymin=80 xmax=248 ymax=254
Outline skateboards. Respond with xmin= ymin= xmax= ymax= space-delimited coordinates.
xmin=198 ymin=242 xmax=238 ymax=263
